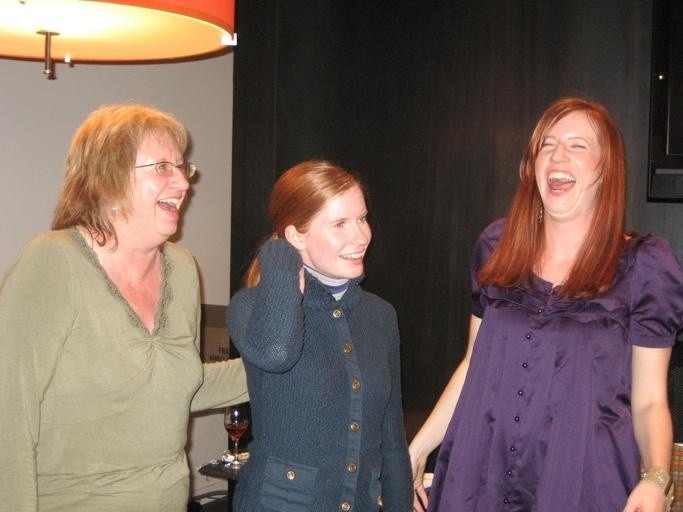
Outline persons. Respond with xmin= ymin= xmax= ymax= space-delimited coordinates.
xmin=407 ymin=96 xmax=683 ymax=512
xmin=225 ymin=159 xmax=415 ymax=512
xmin=1 ymin=102 xmax=251 ymax=512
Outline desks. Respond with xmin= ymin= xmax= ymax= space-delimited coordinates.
xmin=198 ymin=449 xmax=247 ymax=512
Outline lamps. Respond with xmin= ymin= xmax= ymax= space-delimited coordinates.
xmin=0 ymin=0 xmax=236 ymax=77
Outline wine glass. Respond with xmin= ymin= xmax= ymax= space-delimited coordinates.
xmin=224 ymin=405 xmax=250 ymax=471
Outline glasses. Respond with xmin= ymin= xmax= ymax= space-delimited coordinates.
xmin=135 ymin=160 xmax=197 ymax=178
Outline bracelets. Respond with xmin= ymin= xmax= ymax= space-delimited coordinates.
xmin=642 ymin=464 xmax=675 ymax=512
